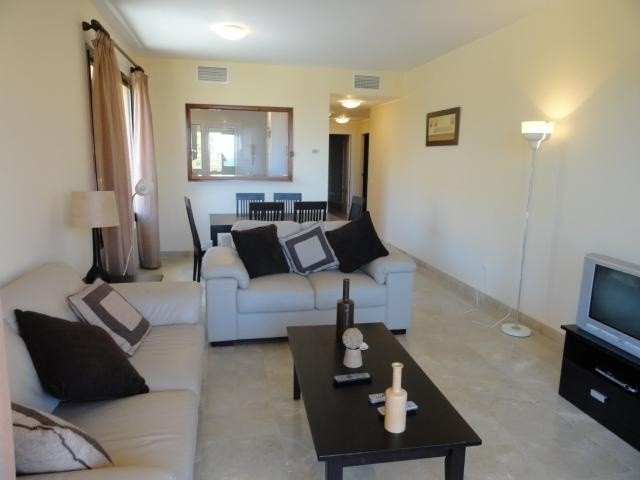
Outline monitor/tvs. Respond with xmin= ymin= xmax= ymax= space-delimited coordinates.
xmin=576 ymin=253 xmax=640 ymax=359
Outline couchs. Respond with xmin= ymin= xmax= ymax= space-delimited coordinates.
xmin=0 ymin=257 xmax=208 ymax=479
xmin=199 ymin=218 xmax=418 ymax=348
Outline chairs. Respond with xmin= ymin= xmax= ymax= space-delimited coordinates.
xmin=184 ymin=189 xmax=368 ymax=281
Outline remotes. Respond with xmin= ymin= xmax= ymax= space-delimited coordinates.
xmin=368 ymin=392 xmax=386 ymax=404
xmin=334 ymin=372 xmax=370 ymax=384
xmin=377 ymin=400 xmax=418 ymax=416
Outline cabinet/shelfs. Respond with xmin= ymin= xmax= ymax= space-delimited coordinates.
xmin=114 ymin=271 xmax=166 ymax=284
xmin=553 ymin=322 xmax=639 ymax=449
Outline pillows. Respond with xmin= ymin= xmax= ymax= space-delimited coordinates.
xmin=65 ymin=277 xmax=154 ymax=358
xmin=11 ymin=302 xmax=155 ymax=406
xmin=325 ymin=211 xmax=391 ymax=272
xmin=8 ymin=395 xmax=111 ymax=474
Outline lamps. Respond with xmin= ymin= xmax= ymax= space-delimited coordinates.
xmin=334 ymin=114 xmax=351 ymax=125
xmin=339 ymin=99 xmax=362 ymax=110
xmin=250 ymin=143 xmax=256 ymax=166
xmin=496 ymin=118 xmax=555 ymax=338
xmin=66 ymin=189 xmax=123 ymax=281
xmin=209 ymin=21 xmax=251 ymax=41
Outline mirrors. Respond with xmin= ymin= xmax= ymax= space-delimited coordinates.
xmin=184 ymin=102 xmax=294 ymax=181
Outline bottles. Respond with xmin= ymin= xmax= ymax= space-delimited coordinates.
xmin=342 ymin=348 xmax=363 ymax=369
xmin=383 ymin=362 xmax=409 ymax=434
xmin=336 ymin=278 xmax=355 ymax=339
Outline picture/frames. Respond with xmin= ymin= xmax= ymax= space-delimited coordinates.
xmin=423 ymin=107 xmax=462 ymax=147
